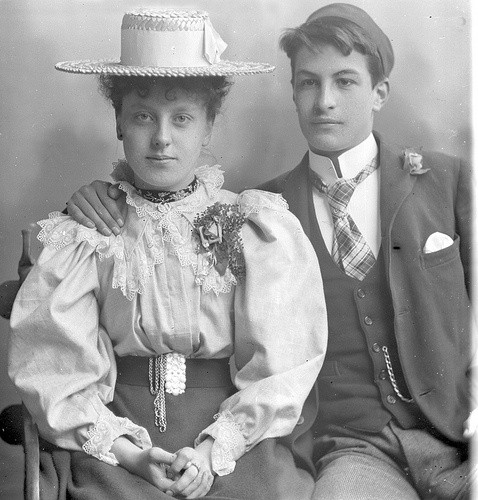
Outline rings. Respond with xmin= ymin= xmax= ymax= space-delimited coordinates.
xmin=191 ymin=462 xmax=201 ymax=472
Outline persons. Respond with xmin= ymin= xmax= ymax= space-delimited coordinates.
xmin=64 ymin=3 xmax=474 ymax=500
xmin=8 ymin=6 xmax=329 ymax=500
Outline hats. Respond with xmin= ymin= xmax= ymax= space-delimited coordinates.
xmin=54 ymin=11 xmax=277 ymax=79
xmin=307 ymin=4 xmax=393 ymax=79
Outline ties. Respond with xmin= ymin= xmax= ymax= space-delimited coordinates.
xmin=308 ymin=153 xmax=381 ymax=281
